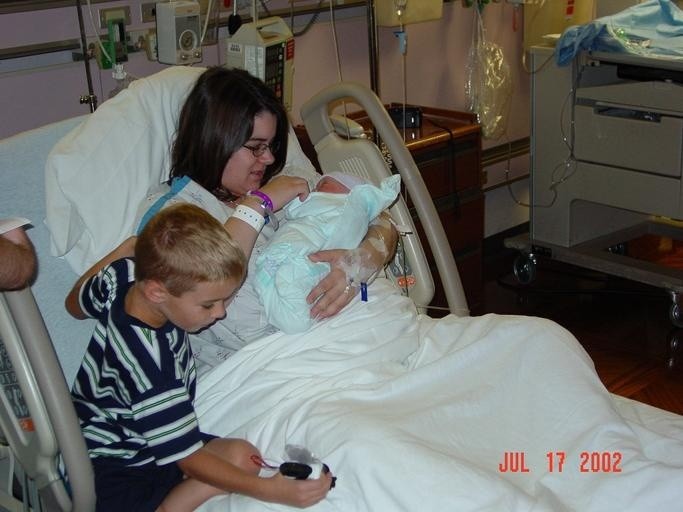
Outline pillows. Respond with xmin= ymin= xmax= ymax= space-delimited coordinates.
xmin=41 ymin=64 xmax=320 ymax=275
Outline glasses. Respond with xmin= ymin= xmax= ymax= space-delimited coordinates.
xmin=242 ymin=141 xmax=281 ymax=158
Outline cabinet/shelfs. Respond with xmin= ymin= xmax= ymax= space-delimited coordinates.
xmin=527 ymin=43 xmax=683 ymax=297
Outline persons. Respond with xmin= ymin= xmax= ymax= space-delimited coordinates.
xmin=308 ymin=171 xmax=363 ymax=197
xmin=60 ymin=200 xmax=340 ymax=512
xmin=123 ymin=62 xmax=401 ymax=363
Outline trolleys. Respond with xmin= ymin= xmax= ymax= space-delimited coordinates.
xmin=510 ymin=29 xmax=683 ymax=327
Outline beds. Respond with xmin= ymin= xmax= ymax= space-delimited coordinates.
xmin=0 ymin=86 xmax=683 ymax=512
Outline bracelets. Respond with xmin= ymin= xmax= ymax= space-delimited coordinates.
xmin=229 ymin=203 xmax=266 ymax=232
xmin=250 ymin=190 xmax=274 ymax=212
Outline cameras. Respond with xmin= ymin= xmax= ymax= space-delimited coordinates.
xmin=389 ymin=107 xmax=423 ymax=128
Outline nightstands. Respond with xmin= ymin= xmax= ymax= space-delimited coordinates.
xmin=294 ymin=103 xmax=486 ymax=317
